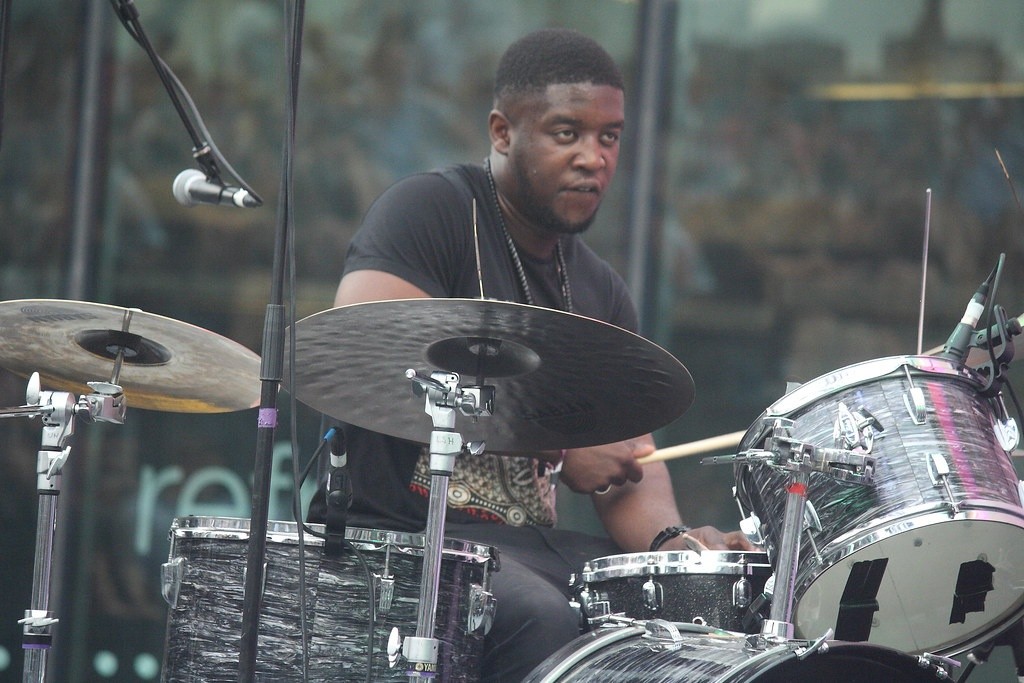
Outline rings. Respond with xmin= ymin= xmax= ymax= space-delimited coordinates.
xmin=594 ymin=484 xmax=612 ymax=495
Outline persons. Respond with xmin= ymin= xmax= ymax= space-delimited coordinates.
xmin=307 ymin=26 xmax=757 ymax=683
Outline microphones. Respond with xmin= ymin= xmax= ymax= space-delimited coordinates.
xmin=173 ymin=169 xmax=257 ymax=211
xmin=939 ymin=283 xmax=990 ymax=361
xmin=323 ymin=430 xmax=346 ymax=558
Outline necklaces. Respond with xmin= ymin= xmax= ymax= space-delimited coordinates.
xmin=483 ymin=155 xmax=575 ymax=315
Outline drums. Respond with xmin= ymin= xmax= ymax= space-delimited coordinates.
xmin=568 ymin=550 xmax=771 ymax=637
xmin=521 ymin=613 xmax=952 ymax=682
xmin=160 ymin=518 xmax=500 ymax=682
xmin=732 ymin=356 xmax=1024 ymax=657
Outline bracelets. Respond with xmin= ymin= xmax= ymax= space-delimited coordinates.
xmin=650 ymin=525 xmax=690 ymax=552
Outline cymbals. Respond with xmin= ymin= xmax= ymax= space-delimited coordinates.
xmin=283 ymin=298 xmax=695 ymax=455
xmin=2 ymin=300 xmax=281 ymax=412
